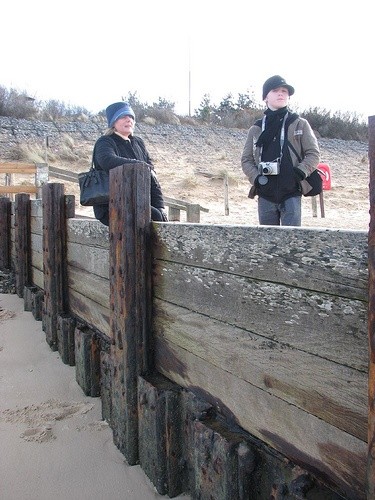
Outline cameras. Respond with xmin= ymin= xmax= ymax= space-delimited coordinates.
xmin=257 ymin=161 xmax=280 ymax=176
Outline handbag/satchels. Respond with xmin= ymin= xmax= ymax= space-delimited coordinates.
xmin=77 ymin=136 xmax=120 ymax=206
xmin=301 ymin=169 xmax=323 ymax=196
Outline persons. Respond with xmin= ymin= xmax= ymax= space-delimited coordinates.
xmin=92 ymin=102 xmax=170 ymax=227
xmin=240 ymin=75 xmax=322 ymax=227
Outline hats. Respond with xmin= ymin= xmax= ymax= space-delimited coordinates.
xmin=262 ymin=76 xmax=295 ymax=99
xmin=106 ymin=102 xmax=136 ymax=129
